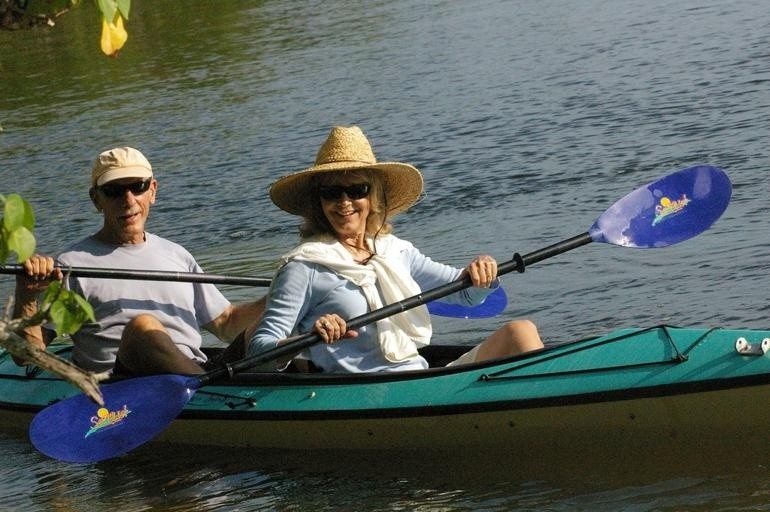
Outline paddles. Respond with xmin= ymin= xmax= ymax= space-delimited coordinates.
xmin=0 ymin=261 xmax=509 ymax=320
xmin=26 ymin=165 xmax=732 ymax=468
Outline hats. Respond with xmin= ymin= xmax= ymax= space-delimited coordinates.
xmin=271 ymin=125 xmax=423 ymax=219
xmin=92 ymin=147 xmax=152 ymax=187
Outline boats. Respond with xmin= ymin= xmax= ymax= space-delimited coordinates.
xmin=0 ymin=314 xmax=770 ymax=456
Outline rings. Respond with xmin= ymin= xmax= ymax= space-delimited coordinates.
xmin=322 ymin=321 xmax=329 ymax=329
xmin=487 ymin=272 xmax=492 ymax=278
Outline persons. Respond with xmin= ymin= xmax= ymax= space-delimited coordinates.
xmin=242 ymin=123 xmax=546 ymax=377
xmin=9 ymin=145 xmax=279 ymax=392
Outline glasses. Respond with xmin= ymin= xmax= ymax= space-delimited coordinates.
xmin=321 ymin=182 xmax=371 ymax=200
xmin=102 ymin=177 xmax=151 ymax=196
